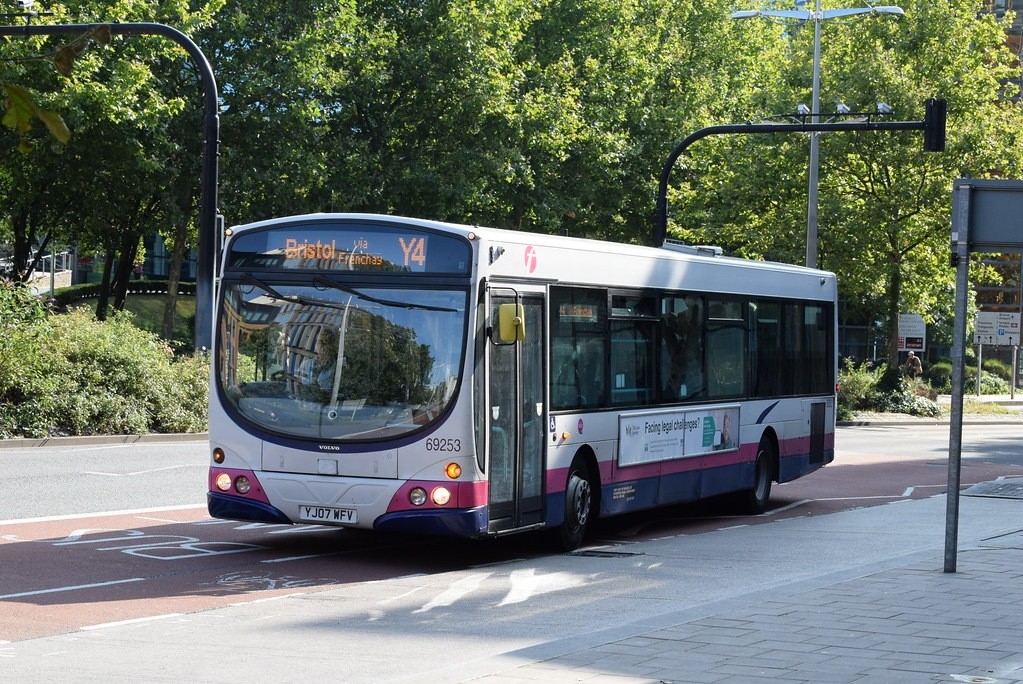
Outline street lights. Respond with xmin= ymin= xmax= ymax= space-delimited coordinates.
xmin=730 ymin=1 xmax=904 ymax=269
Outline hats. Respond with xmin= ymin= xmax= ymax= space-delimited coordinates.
xmin=907 ymin=350 xmax=915 ymax=356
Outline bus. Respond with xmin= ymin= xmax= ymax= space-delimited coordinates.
xmin=207 ymin=212 xmax=840 ymax=550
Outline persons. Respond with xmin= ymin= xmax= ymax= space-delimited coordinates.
xmin=297 ymin=329 xmax=335 ymax=391
xmin=575 ymin=296 xmax=737 ymax=402
xmin=906 ymin=351 xmax=923 ymax=380
